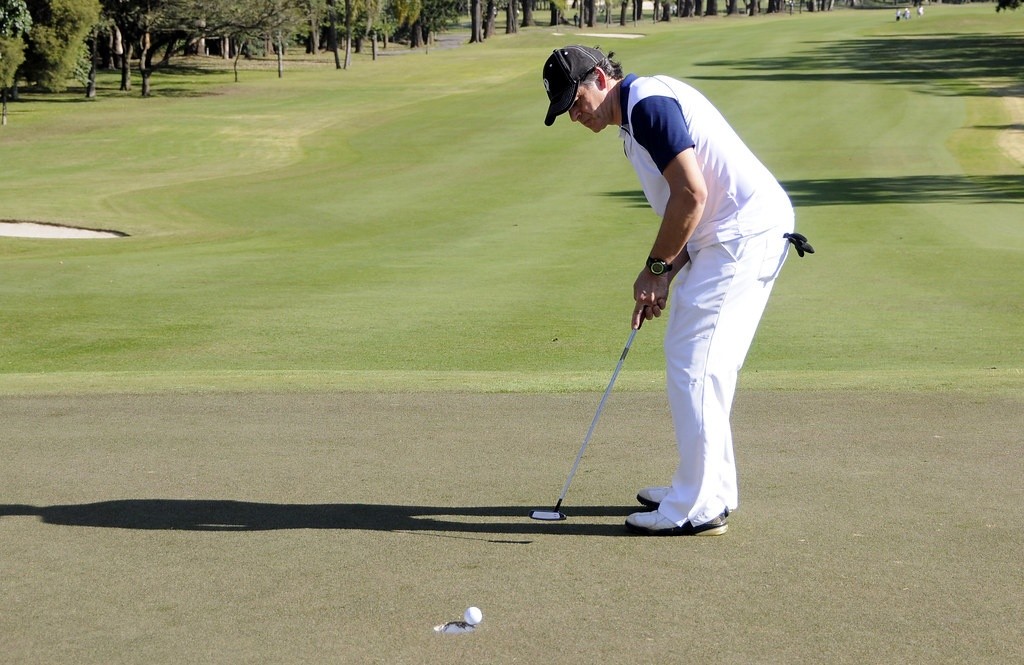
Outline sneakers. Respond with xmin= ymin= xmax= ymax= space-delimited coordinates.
xmin=624 ymin=507 xmax=731 ymax=535
xmin=638 ymin=486 xmax=672 ymax=509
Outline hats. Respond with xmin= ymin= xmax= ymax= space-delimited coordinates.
xmin=543 ymin=44 xmax=606 ymax=126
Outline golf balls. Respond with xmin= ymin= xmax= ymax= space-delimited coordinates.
xmin=464 ymin=606 xmax=483 ymax=626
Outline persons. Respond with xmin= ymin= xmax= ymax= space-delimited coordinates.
xmin=895 ymin=9 xmax=900 ymax=21
xmin=918 ymin=6 xmax=923 ymax=16
xmin=544 ymin=45 xmax=795 ymax=536
xmin=904 ymin=9 xmax=910 ymax=20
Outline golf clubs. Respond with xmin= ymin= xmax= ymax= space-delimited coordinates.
xmin=527 ymin=305 xmax=646 ymax=520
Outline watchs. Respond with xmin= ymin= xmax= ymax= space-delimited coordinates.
xmin=646 ymin=256 xmax=673 ymax=275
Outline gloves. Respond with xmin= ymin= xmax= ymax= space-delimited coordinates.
xmin=783 ymin=232 xmax=814 ymax=258
